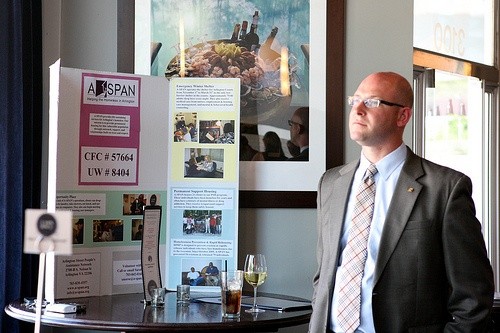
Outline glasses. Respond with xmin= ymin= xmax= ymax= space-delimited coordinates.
xmin=348 ymin=95 xmax=404 ymax=109
xmin=288 ymin=120 xmax=301 ymax=127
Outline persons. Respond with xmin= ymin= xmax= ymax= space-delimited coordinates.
xmin=190 ymin=152 xmax=214 ymax=172
xmin=72 ymin=219 xmax=83 ymax=244
xmin=203 ymin=261 xmax=219 ymax=286
xmin=197 ymin=120 xmax=235 ymax=144
xmin=308 ymin=72 xmax=500 ymax=333
xmin=237 ymin=106 xmax=309 ymax=162
xmin=92 ymin=219 xmax=123 ymax=241
xmin=149 ymin=194 xmax=157 ymax=205
xmin=183 ymin=212 xmax=222 ymax=234
xmin=123 ymin=194 xmax=147 ymax=216
xmin=187 ymin=267 xmax=205 ymax=287
xmin=174 ymin=116 xmax=195 ymax=142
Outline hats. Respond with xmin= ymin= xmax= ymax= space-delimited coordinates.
xmin=75 ymin=219 xmax=84 ymax=225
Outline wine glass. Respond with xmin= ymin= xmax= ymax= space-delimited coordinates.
xmin=271 ymin=72 xmax=302 ymax=97
xmin=184 ymin=37 xmax=198 ymax=56
xmin=197 ymin=34 xmax=211 ymax=52
xmin=272 ymin=63 xmax=303 ymax=79
xmin=243 ymin=254 xmax=267 ymax=313
xmin=171 ymin=42 xmax=191 ymax=67
xmin=271 ymin=48 xmax=297 ymax=68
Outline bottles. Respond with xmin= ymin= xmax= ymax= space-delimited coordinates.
xmin=239 ymin=21 xmax=248 ymax=40
xmin=445 ymin=99 xmax=457 ymax=139
xmin=460 ymin=103 xmax=468 ymax=138
xmin=242 ymin=11 xmax=259 ymax=52
xmin=231 ymin=24 xmax=241 ymax=40
xmin=427 ymin=106 xmax=444 ymax=140
xmin=258 ymin=25 xmax=279 ymax=60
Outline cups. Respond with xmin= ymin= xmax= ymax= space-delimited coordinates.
xmin=150 ymin=288 xmax=165 ymax=307
xmin=177 ymin=285 xmax=190 ymax=304
xmin=220 ymin=270 xmax=244 ymax=318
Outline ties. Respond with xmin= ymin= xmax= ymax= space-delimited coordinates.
xmin=334 ymin=164 xmax=378 ymax=333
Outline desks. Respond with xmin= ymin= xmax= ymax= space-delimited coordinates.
xmin=4 ymin=291 xmax=313 ymax=333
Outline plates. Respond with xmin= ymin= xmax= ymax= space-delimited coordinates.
xmin=166 ymin=39 xmax=291 ymax=116
